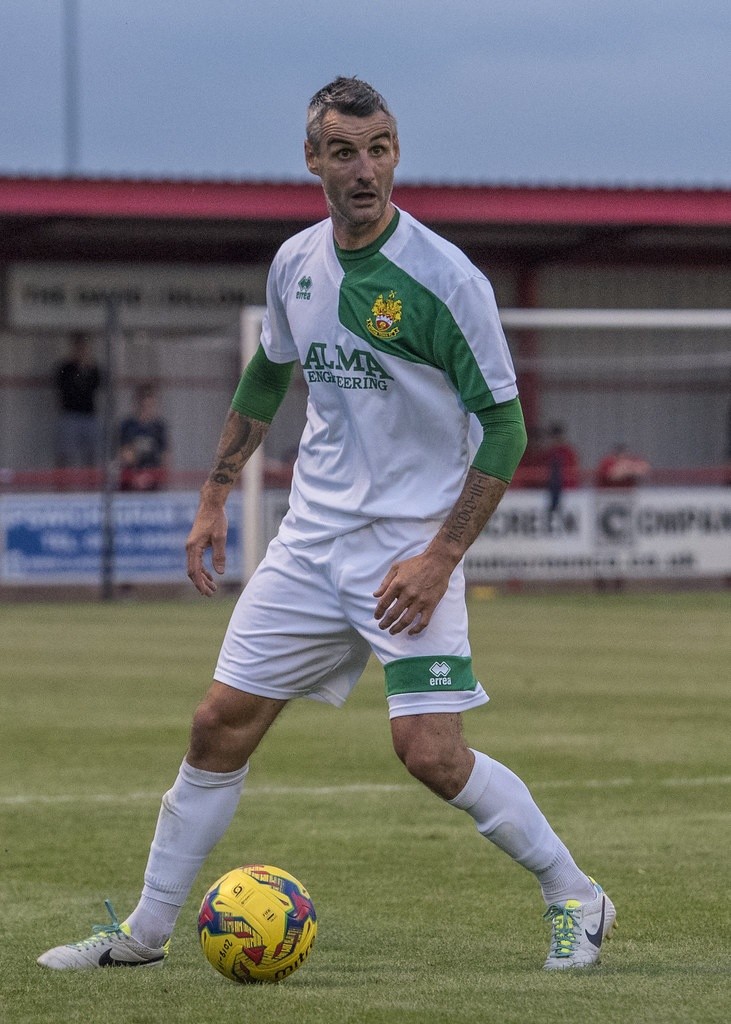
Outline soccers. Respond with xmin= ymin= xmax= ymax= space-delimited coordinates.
xmin=195 ymin=862 xmax=320 ymax=986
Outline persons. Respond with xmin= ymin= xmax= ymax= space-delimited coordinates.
xmin=114 ymin=384 xmax=170 ymax=492
xmin=537 ymin=424 xmax=574 ymax=511
xmin=49 ymin=335 xmax=107 ymax=489
xmin=596 ymin=443 xmax=649 ymax=487
xmin=38 ymin=78 xmax=618 ymax=969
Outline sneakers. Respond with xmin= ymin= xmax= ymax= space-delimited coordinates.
xmin=37 ymin=899 xmax=166 ymax=969
xmin=541 ymin=876 xmax=615 ymax=971
